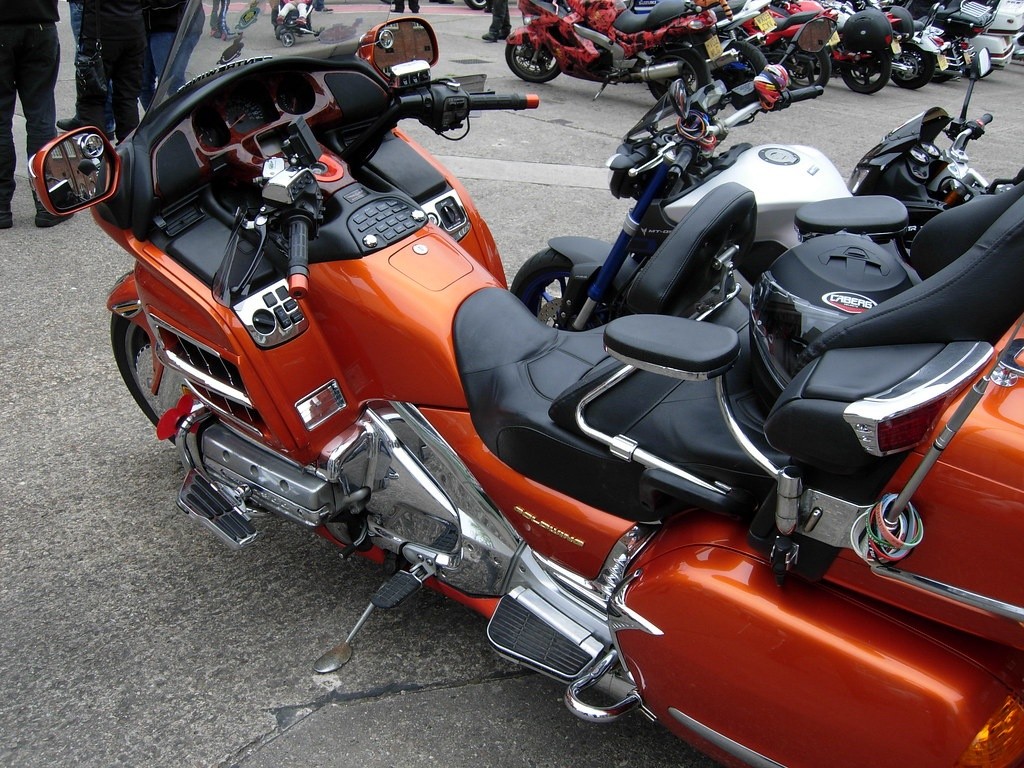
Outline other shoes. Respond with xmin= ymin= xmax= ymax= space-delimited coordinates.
xmin=412 ymin=5 xmax=419 ymax=13
xmin=497 ymin=30 xmax=511 ymax=40
xmin=56 ymin=119 xmax=114 ymax=140
xmin=484 ymin=9 xmax=492 ymax=13
xmin=0 ymin=208 xmax=13 ymax=229
xmin=482 ymin=33 xmax=497 ymax=42
xmin=438 ymin=0 xmax=454 ymax=4
xmin=391 ymin=10 xmax=403 ymax=13
xmin=35 ymin=203 xmax=74 ymax=227
xmin=277 ymin=15 xmax=284 ymax=24
xmin=315 ymin=7 xmax=333 ymax=13
xmin=296 ymin=18 xmax=306 ymax=25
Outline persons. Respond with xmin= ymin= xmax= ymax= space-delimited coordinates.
xmin=210 ymin=0 xmax=512 ymax=44
xmin=0 ymin=0 xmax=207 ymax=229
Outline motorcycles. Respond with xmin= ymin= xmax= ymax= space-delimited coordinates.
xmin=824 ymin=1 xmax=954 ymax=92
xmin=815 ymin=3 xmax=897 ymax=95
xmin=712 ymin=3 xmax=781 ymax=112
xmin=721 ymin=4 xmax=838 ymax=99
xmin=506 ymin=1 xmax=721 ymax=103
xmin=842 ymin=48 xmax=1021 ymax=210
xmin=30 ymin=16 xmax=1024 ymax=768
xmin=959 ymin=1 xmax=1023 ymax=78
xmin=508 ymin=71 xmax=914 ymax=332
xmin=889 ymin=2 xmax=998 ymax=87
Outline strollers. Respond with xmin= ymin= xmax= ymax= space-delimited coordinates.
xmin=271 ymin=1 xmax=322 ymax=47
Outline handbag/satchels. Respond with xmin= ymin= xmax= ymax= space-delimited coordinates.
xmin=75 ymin=52 xmax=108 ymax=96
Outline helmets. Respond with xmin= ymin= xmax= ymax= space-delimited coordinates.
xmin=749 ymin=234 xmax=914 ymax=419
